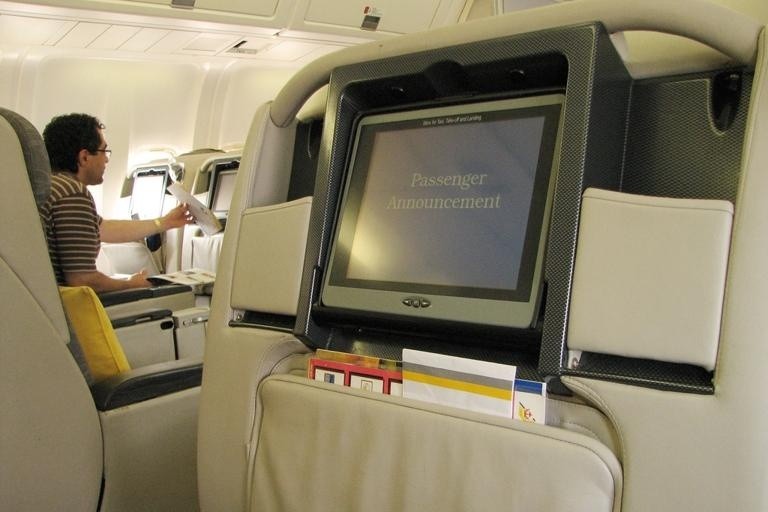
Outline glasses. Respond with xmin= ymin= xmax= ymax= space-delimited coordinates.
xmin=97 ymin=150 xmax=112 ymax=160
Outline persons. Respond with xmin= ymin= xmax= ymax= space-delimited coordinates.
xmin=39 ymin=113 xmax=196 ymax=293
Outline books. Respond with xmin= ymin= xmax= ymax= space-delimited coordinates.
xmin=147 ymin=267 xmax=217 ymax=287
xmin=301 ymin=343 xmax=548 ymax=428
xmin=165 ymin=183 xmax=222 ymax=238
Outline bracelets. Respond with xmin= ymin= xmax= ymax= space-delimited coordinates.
xmin=152 ymin=219 xmax=161 ymax=235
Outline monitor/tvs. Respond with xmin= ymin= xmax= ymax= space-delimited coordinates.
xmin=130 ymin=169 xmax=168 ymax=219
xmin=210 ymin=169 xmax=238 ymax=219
xmin=321 ymin=92 xmax=566 ymax=330
